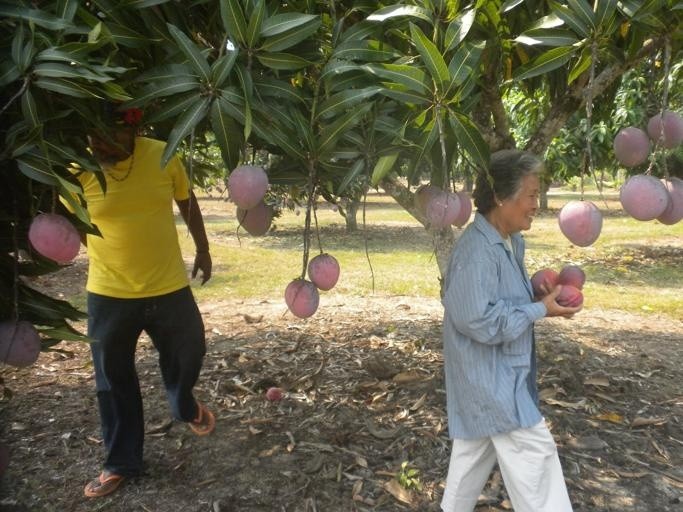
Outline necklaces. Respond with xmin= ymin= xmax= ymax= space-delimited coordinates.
xmin=101 ymin=148 xmax=135 ymax=182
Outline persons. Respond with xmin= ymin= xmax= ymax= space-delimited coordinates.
xmin=437 ymin=145 xmax=576 ymax=512
xmin=54 ymin=119 xmax=217 ymax=500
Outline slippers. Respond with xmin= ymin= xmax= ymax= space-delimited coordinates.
xmin=188 ymin=398 xmax=215 ymax=436
xmin=84 ymin=470 xmax=125 ymax=499
